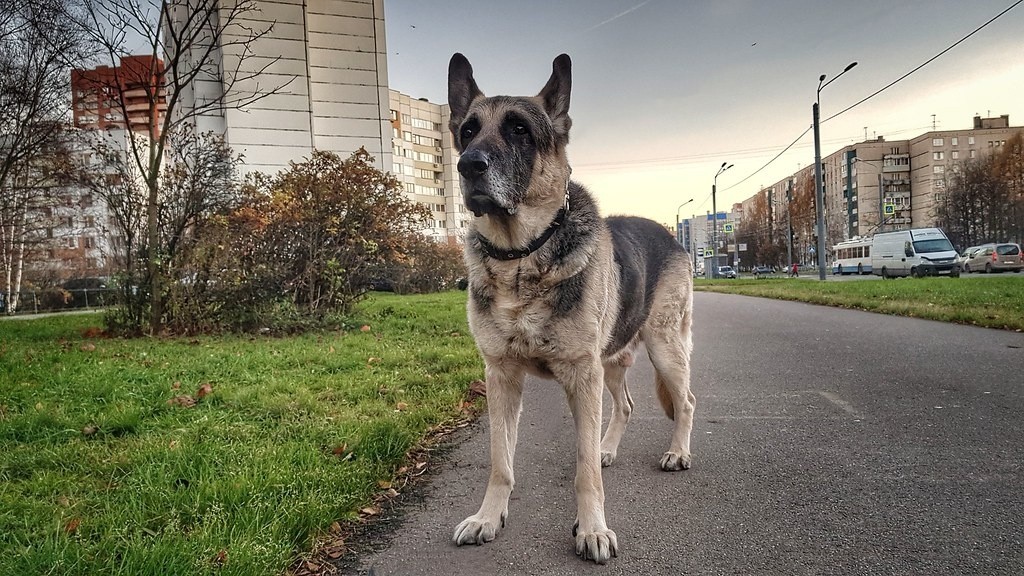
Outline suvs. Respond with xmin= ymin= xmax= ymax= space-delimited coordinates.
xmin=752 ymin=264 xmax=775 ymax=274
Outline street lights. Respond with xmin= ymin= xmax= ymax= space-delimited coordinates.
xmin=813 ymin=61 xmax=859 ymax=279
xmin=712 ymin=161 xmax=734 ymax=278
xmin=676 ymin=199 xmax=695 ymax=241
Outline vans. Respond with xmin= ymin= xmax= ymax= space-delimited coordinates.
xmin=782 ymin=262 xmax=806 ymax=273
xmin=961 ymin=242 xmax=1024 ymax=273
xmin=712 ymin=265 xmax=737 ymax=278
xmin=872 ymin=227 xmax=960 ymax=277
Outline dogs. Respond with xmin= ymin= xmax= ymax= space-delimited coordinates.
xmin=448 ymin=51 xmax=697 ymax=564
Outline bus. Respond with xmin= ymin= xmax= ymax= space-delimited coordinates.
xmin=830 ymin=236 xmax=871 ymax=275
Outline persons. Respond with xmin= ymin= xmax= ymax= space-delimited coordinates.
xmin=791 ymin=263 xmax=798 ymax=277
xmin=752 ymin=265 xmax=758 ymax=280
